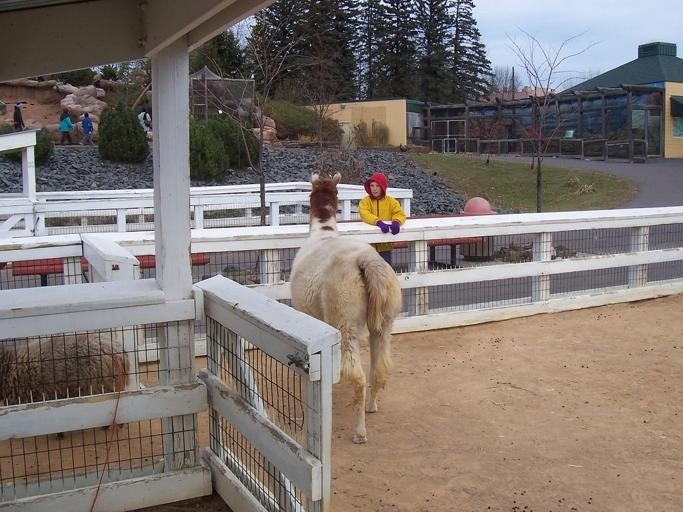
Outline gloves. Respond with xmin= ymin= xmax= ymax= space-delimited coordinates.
xmin=376 ymin=220 xmax=390 ymax=233
xmin=390 ymin=220 xmax=400 ymax=235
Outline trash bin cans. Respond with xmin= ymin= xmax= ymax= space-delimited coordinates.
xmin=460 ymin=196 xmax=496 ymax=261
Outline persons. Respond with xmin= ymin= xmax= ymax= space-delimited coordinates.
xmin=138 ymin=108 xmax=152 ymax=137
xmin=14 ymin=102 xmax=26 ymax=131
xmin=359 ymin=173 xmax=407 ymax=265
xmin=80 ymin=113 xmax=95 ymax=145
xmin=59 ymin=109 xmax=73 ymax=146
